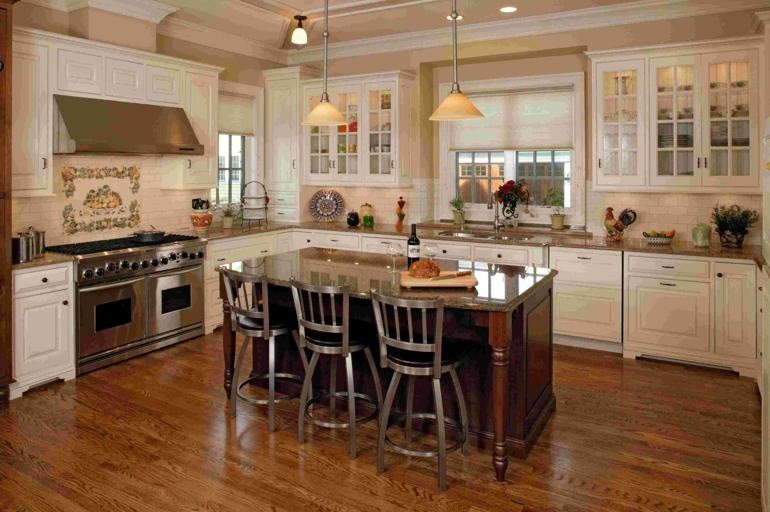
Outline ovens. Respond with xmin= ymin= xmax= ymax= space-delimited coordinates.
xmin=75 ymin=238 xmax=208 ymax=379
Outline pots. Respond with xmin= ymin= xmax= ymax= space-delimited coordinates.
xmin=26 ymin=227 xmax=45 ymax=261
xmin=130 ymin=230 xmax=168 ymax=241
xmin=12 ymin=233 xmax=27 ymax=263
xmin=132 ymin=229 xmax=166 ymax=243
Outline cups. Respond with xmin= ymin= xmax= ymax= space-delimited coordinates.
xmin=385 ymin=241 xmax=402 ymax=276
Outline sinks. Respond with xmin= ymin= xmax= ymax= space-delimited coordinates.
xmin=494 ymin=234 xmax=533 ymax=240
xmin=439 ymin=230 xmax=494 ymax=240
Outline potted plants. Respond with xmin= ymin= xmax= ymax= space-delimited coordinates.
xmin=222 ymin=203 xmax=243 ymax=228
xmin=540 ymin=187 xmax=567 ymax=231
xmin=709 ymin=200 xmax=759 ymax=250
xmin=449 ymin=196 xmax=468 ymax=227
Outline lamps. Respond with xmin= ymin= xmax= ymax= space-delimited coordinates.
xmin=427 ymin=1 xmax=485 ymax=123
xmin=301 ymin=0 xmax=349 ymax=126
xmin=290 ymin=14 xmax=308 ymax=45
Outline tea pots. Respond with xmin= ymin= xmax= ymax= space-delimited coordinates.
xmin=612 ymin=75 xmax=633 ymax=95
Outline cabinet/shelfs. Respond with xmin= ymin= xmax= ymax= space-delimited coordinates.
xmin=12 ymin=25 xmax=56 ymax=198
xmin=420 ymin=237 xmax=547 ymax=269
xmin=624 ymin=252 xmax=712 ymax=368
xmin=592 ymin=45 xmax=649 ymax=193
xmin=204 ymin=229 xmax=293 ymax=335
xmin=263 ymin=67 xmax=320 ymax=223
xmin=549 ymin=247 xmax=623 ymax=354
xmin=10 ymin=251 xmax=76 ymax=400
xmin=294 ymin=227 xmax=409 ymax=254
xmin=713 ymin=259 xmax=758 ymax=381
xmin=301 ymin=74 xmax=363 ymax=187
xmin=160 ymin=53 xmax=226 ymax=190
xmin=263 ymin=186 xmax=320 ymax=223
xmin=56 ymin=33 xmax=182 ymax=109
xmin=363 ymin=69 xmax=416 ymax=187
xmin=649 ymin=36 xmax=762 ymax=195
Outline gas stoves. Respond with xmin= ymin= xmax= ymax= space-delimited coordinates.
xmin=46 ymin=230 xmax=199 ymax=257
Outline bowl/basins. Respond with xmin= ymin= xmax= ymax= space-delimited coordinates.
xmin=712 ymin=126 xmax=721 ymax=146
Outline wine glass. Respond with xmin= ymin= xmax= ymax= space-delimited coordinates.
xmin=422 ymin=243 xmax=439 ymax=271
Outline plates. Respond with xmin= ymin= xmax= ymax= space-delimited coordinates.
xmin=659 ymin=134 xmax=693 ymax=148
xmin=646 ymin=236 xmax=673 ymax=245
xmin=309 ymin=188 xmax=344 ymax=223
xmin=243 ymin=206 xmax=265 ymax=210
xmin=732 ymin=137 xmax=749 ymax=146
xmin=242 ymin=218 xmax=268 ymax=221
xmin=243 ymin=196 xmax=264 ymax=199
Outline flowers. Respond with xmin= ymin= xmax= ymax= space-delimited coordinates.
xmin=494 ymin=178 xmax=536 ymax=213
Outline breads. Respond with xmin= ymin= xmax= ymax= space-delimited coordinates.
xmin=410 ymin=261 xmax=439 ymax=277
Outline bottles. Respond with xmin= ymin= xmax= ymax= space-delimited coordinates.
xmin=363 ymin=205 xmax=375 ymax=227
xmin=408 ymin=225 xmax=420 ymax=266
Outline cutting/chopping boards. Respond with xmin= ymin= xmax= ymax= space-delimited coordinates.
xmin=399 ymin=271 xmax=479 ymax=287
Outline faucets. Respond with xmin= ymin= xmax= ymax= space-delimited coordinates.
xmin=487 ymin=192 xmax=505 ymax=231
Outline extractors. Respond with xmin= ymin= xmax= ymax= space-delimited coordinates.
xmin=52 ymin=93 xmax=205 ymax=155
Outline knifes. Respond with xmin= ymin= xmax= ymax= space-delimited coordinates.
xmin=430 ymin=270 xmax=472 ymax=281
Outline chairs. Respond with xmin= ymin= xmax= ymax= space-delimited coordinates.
xmin=287 ymin=276 xmax=384 ymax=458
xmin=221 ymin=269 xmax=313 ymax=433
xmin=367 ymin=288 xmax=473 ymax=490
